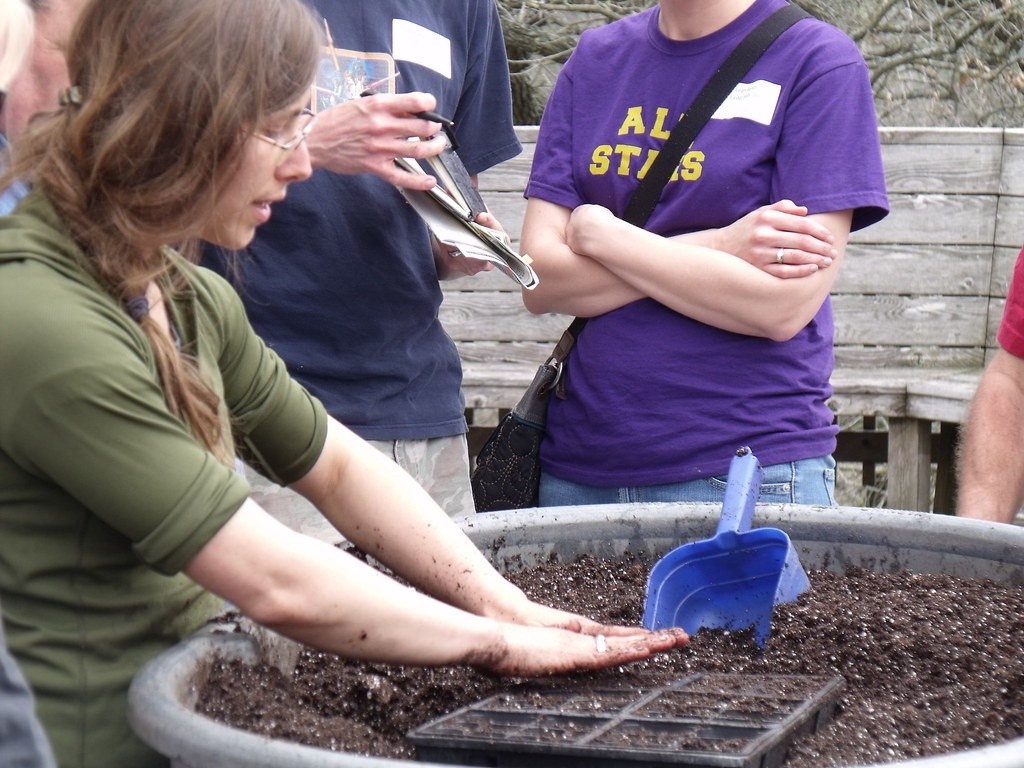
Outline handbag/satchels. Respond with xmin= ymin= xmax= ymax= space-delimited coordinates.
xmin=472 ymin=319 xmax=583 ymax=514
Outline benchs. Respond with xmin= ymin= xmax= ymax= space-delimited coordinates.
xmin=433 ymin=126 xmax=1024 ymax=509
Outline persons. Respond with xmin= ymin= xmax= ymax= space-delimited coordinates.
xmin=520 ymin=0 xmax=891 ymax=504
xmin=197 ymin=0 xmax=524 ymax=546
xmin=956 ymin=247 xmax=1024 ymax=527
xmin=0 ymin=0 xmax=690 ymax=768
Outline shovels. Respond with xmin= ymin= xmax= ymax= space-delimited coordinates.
xmin=639 ymin=443 xmax=815 ymax=658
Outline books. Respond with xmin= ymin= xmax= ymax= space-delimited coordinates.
xmin=391 ymin=128 xmax=488 ymax=222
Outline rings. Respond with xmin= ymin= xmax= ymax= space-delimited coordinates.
xmin=449 ymin=251 xmax=461 ymax=257
xmin=776 ymin=248 xmax=783 ymax=264
xmin=597 ymin=635 xmax=605 ymax=652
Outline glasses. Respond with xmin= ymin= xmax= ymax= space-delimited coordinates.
xmin=236 ymin=108 xmax=320 ymax=153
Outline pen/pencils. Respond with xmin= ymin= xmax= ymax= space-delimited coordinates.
xmin=359 ymin=90 xmax=456 ymax=129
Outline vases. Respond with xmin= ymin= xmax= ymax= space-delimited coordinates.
xmin=128 ymin=500 xmax=1024 ymax=767
